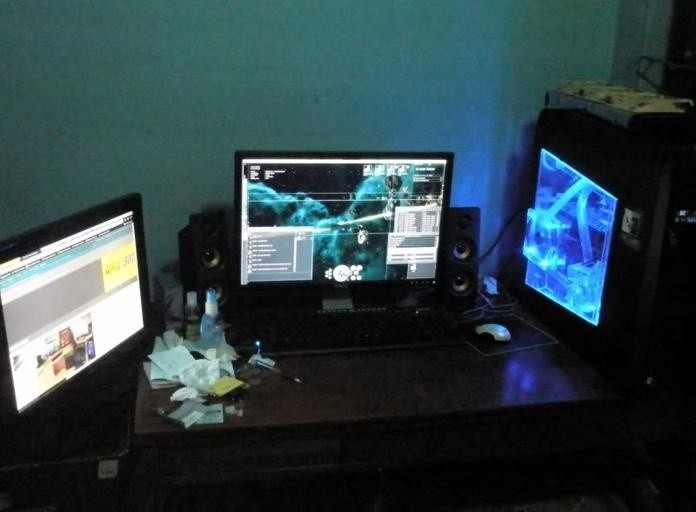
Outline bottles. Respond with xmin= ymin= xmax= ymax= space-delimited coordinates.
xmin=184 ymin=289 xmax=201 ymax=347
xmin=200 ymin=288 xmax=226 ymax=351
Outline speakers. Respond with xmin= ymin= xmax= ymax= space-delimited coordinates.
xmin=176 ymin=208 xmax=232 ymax=317
xmin=450 ymin=207 xmax=480 ymax=312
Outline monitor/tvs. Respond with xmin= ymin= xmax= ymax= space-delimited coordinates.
xmin=0 ymin=190 xmax=154 ymax=422
xmin=233 ymin=149 xmax=453 ymax=310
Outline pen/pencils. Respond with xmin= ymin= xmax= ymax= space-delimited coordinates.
xmin=255 ymin=361 xmax=302 ymax=384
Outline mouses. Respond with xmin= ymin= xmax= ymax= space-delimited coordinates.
xmin=474 ymin=323 xmax=511 ymax=344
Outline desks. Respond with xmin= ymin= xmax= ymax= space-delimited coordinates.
xmin=132 ymin=309 xmax=608 ymax=512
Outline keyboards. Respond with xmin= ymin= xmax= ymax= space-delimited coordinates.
xmin=255 ymin=308 xmax=448 ymax=357
